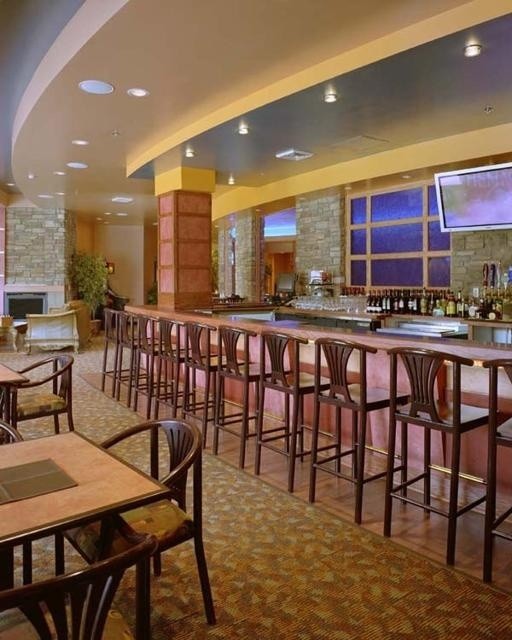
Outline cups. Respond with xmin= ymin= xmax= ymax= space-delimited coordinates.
xmin=294 ymin=295 xmax=343 ymax=312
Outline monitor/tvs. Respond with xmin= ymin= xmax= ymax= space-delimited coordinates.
xmin=432 ymin=164 xmax=512 ymax=233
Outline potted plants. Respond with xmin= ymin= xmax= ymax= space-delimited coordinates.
xmin=66 ymin=246 xmax=109 ymax=337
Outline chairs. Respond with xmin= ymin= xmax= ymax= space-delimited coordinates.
xmin=3 ymin=355 xmax=74 ymax=444
xmin=24 ymin=306 xmax=91 ymax=355
xmin=54 ymin=418 xmax=217 ymax=640
xmin=0 ymin=532 xmax=161 ymax=640
xmin=0 ymin=421 xmax=24 ymax=445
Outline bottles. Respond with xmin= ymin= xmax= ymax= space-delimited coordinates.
xmin=343 ymin=287 xmax=503 ymax=320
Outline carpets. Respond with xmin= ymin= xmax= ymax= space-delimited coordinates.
xmin=0 ymin=325 xmax=512 ymax=640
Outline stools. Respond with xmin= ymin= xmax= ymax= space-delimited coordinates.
xmin=308 ymin=338 xmax=410 ymax=525
xmin=213 ymin=324 xmax=289 ymax=470
xmin=102 ymin=306 xmax=216 ymax=424
xmin=182 ymin=318 xmax=248 ymax=450
xmin=384 ymin=347 xmax=496 ymax=563
xmin=481 ymin=359 xmax=512 ymax=585
xmin=254 ymin=330 xmax=342 ymax=491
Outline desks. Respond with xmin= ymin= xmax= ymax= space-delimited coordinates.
xmin=0 ymin=322 xmax=28 ymax=353
